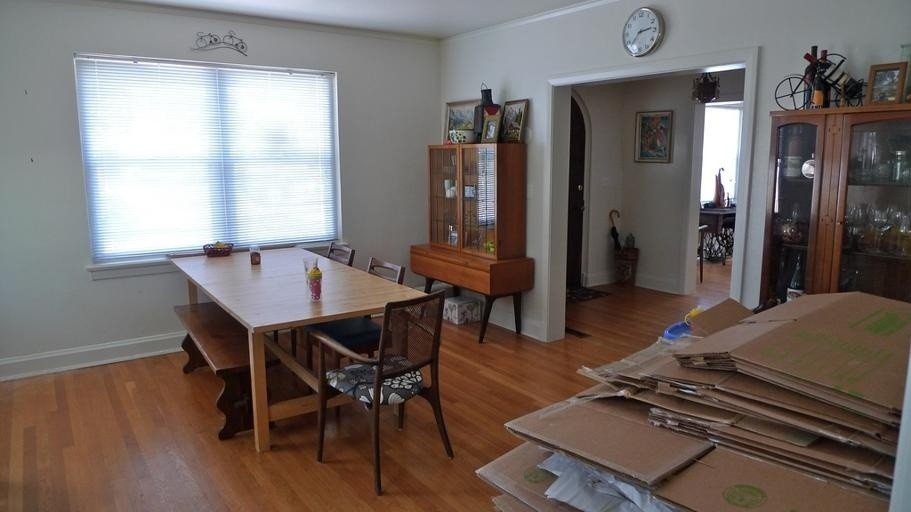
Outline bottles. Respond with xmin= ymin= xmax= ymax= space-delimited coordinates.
xmin=308 ymin=267 xmax=322 ymax=303
xmin=782 ymin=124 xmax=805 ymax=178
xmin=891 ymin=149 xmax=910 ymax=184
xmin=304 ymin=256 xmax=319 ymax=286
xmin=803 ymin=44 xmax=861 ymax=108
xmin=248 ymin=244 xmax=262 ymax=266
xmin=787 ymin=252 xmax=805 ymax=300
xmin=443 ymin=178 xmax=476 ymax=199
xmin=766 ymin=247 xmax=788 ymax=306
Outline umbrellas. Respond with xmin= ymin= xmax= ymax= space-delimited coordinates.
xmin=608 ymin=209 xmax=621 ymax=249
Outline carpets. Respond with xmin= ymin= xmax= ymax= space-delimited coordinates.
xmin=565 ymin=284 xmax=613 ymax=305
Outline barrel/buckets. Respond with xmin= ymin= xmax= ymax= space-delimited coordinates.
xmin=663 ymin=320 xmax=692 ymax=341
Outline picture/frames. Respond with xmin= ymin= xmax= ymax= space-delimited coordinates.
xmin=633 ymin=108 xmax=675 ymax=165
xmin=862 ymin=61 xmax=909 ymax=106
xmin=442 ymin=98 xmax=485 ymax=147
xmin=479 ymin=111 xmax=503 ymax=145
xmin=498 ymin=99 xmax=532 ymax=142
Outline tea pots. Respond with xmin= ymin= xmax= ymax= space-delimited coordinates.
xmin=772 ymin=206 xmax=808 ymax=247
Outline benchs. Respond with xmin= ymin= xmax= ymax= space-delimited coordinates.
xmin=174 ymin=298 xmax=279 ymax=444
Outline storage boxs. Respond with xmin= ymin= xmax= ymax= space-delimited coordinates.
xmin=442 ymin=295 xmax=485 ymax=327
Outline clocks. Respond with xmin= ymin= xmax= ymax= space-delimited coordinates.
xmin=622 ymin=5 xmax=667 ymax=59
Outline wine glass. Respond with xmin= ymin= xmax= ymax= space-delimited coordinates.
xmin=839 ymin=200 xmax=911 ymax=255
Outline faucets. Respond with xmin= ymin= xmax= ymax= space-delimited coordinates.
xmin=718 ymin=167 xmax=725 ymax=177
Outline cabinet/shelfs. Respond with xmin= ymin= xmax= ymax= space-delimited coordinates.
xmin=754 ymin=103 xmax=841 ymax=317
xmin=424 ymin=135 xmax=528 ymax=264
xmin=815 ymin=103 xmax=910 ymax=309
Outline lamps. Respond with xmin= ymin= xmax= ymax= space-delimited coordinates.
xmin=690 ymin=72 xmax=723 ymax=107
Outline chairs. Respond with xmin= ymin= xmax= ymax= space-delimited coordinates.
xmin=306 ymin=257 xmax=405 ymax=416
xmin=696 ymin=222 xmax=710 ymax=284
xmin=274 ymin=242 xmax=356 ymax=358
xmin=316 ymin=289 xmax=455 ymax=496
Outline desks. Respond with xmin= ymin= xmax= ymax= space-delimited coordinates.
xmin=697 ymin=201 xmax=739 ymax=266
xmin=163 ymin=244 xmax=441 ymax=455
xmin=408 ymin=238 xmax=538 ymax=344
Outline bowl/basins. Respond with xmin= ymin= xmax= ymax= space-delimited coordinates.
xmin=449 ymin=128 xmax=478 ymax=144
